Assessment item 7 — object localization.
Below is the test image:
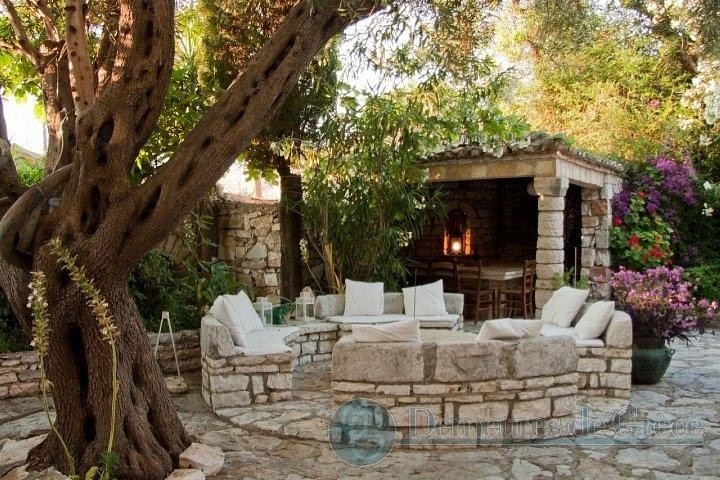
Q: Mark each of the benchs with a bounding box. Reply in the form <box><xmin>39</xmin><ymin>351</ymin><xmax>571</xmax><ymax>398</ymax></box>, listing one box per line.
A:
<box><xmin>329</xmin><ymin>322</ymin><xmax>582</xmax><ymax>424</ymax></box>
<box><xmin>201</xmin><ymin>290</ymin><xmax>302</xmax><ymax>410</ymax></box>
<box><xmin>537</xmin><ymin>293</ymin><xmax>635</xmax><ymax>399</ymax></box>
<box><xmin>317</xmin><ymin>277</ymin><xmax>466</xmax><ymax>335</ymax></box>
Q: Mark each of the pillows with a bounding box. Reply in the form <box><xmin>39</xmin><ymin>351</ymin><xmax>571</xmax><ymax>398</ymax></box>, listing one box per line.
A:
<box><xmin>572</xmin><ymin>301</ymin><xmax>617</xmax><ymax>339</ymax></box>
<box><xmin>542</xmin><ymin>282</ymin><xmax>589</xmax><ymax>327</ymax></box>
<box><xmin>402</xmin><ymin>278</ymin><xmax>450</xmax><ymax>316</ymax></box>
<box><xmin>341</xmin><ymin>274</ymin><xmax>385</xmax><ymax>319</ymax></box>
<box><xmin>208</xmin><ymin>293</ymin><xmax>248</xmax><ymax>347</ymax></box>
<box><xmin>349</xmin><ymin>318</ymin><xmax>425</xmax><ymax>343</ymax></box>
<box><xmin>475</xmin><ymin>314</ymin><xmax>545</xmax><ymax>341</ymax></box>
<box><xmin>222</xmin><ymin>289</ymin><xmax>262</xmax><ymax>332</ymax></box>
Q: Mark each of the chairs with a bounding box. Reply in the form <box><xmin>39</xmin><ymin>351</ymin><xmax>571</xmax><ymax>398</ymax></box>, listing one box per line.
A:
<box><xmin>409</xmin><ymin>252</ymin><xmax>536</xmax><ymax>320</ymax></box>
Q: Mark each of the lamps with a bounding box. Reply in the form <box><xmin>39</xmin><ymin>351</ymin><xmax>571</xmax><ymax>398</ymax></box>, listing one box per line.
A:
<box><xmin>444</xmin><ymin>226</ymin><xmax>468</xmax><ymax>257</ymax></box>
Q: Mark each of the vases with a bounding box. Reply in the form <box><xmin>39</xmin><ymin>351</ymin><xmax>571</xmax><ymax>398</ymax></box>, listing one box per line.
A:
<box><xmin>630</xmin><ymin>345</ymin><xmax>676</xmax><ymax>385</ymax></box>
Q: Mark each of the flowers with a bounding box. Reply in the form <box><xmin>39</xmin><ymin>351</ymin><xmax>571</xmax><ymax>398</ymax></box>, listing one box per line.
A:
<box><xmin>591</xmin><ymin>255</ymin><xmax>717</xmax><ymax>348</ymax></box>
<box><xmin>610</xmin><ymin>189</ymin><xmax>666</xmax><ymax>259</ymax></box>
